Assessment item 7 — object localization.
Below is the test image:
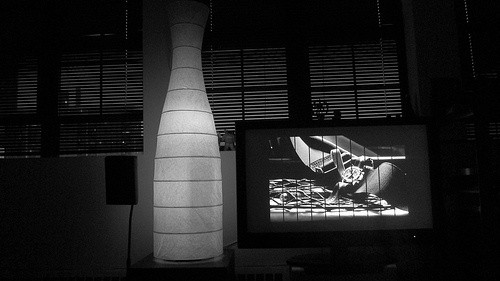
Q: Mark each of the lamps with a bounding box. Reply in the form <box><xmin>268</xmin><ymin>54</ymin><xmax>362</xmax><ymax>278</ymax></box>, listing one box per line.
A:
<box><xmin>151</xmin><ymin>1</ymin><xmax>224</xmax><ymax>262</ymax></box>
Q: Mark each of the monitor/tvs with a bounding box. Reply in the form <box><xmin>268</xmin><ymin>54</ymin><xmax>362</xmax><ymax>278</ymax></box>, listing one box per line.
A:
<box><xmin>236</xmin><ymin>116</ymin><xmax>445</xmax><ymax>249</ymax></box>
